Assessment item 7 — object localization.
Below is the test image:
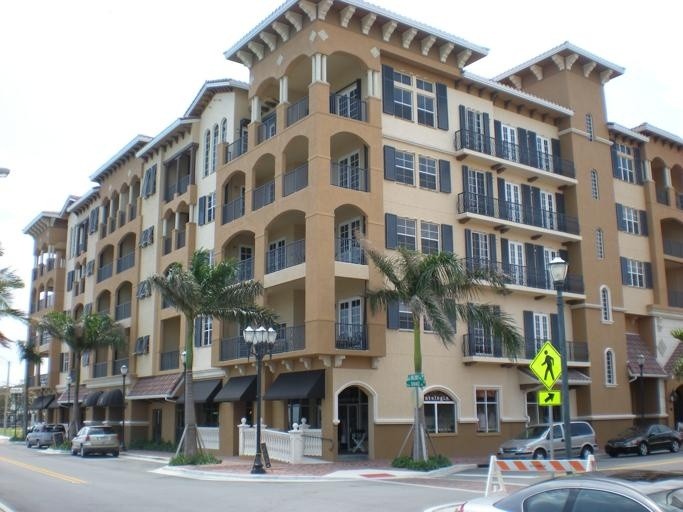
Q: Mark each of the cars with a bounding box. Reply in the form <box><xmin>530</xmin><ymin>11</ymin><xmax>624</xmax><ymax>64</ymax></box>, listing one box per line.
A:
<box><xmin>421</xmin><ymin>468</ymin><xmax>683</xmax><ymax>512</ymax></box>
<box><xmin>604</xmin><ymin>422</ymin><xmax>682</xmax><ymax>457</ymax></box>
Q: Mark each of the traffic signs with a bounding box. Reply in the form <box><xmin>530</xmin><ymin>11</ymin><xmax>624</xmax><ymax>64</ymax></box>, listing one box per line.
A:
<box><xmin>406</xmin><ymin>380</ymin><xmax>426</xmax><ymax>387</ymax></box>
<box><xmin>407</xmin><ymin>373</ymin><xmax>423</xmax><ymax>380</ymax></box>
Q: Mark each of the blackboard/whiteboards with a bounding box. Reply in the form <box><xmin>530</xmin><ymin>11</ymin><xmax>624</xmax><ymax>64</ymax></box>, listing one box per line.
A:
<box><xmin>52</xmin><ymin>432</ymin><xmax>64</xmax><ymax>448</ymax></box>
<box><xmin>260</xmin><ymin>442</ymin><xmax>271</xmax><ymax>468</ymax></box>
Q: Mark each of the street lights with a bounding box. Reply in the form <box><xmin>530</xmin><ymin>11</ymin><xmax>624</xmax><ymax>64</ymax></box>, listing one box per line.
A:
<box><xmin>242</xmin><ymin>324</ymin><xmax>277</xmax><ymax>473</ymax></box>
<box><xmin>636</xmin><ymin>353</ymin><xmax>645</xmax><ymax>422</ymax></box>
<box><xmin>546</xmin><ymin>255</ymin><xmax>576</xmax><ymax>476</ymax></box>
<box><xmin>120</xmin><ymin>364</ymin><xmax>128</xmax><ymax>449</ymax></box>
<box><xmin>40</xmin><ymin>381</ymin><xmax>44</xmax><ymax>423</ymax></box>
<box><xmin>65</xmin><ymin>376</ymin><xmax>70</xmax><ymax>440</ymax></box>
<box><xmin>180</xmin><ymin>350</ymin><xmax>186</xmax><ymax>408</ymax></box>
<box><xmin>13</xmin><ymin>389</ymin><xmax>17</xmax><ymax>439</ymax></box>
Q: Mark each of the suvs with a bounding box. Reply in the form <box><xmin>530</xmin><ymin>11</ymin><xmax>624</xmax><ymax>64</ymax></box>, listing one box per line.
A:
<box><xmin>70</xmin><ymin>425</ymin><xmax>120</xmax><ymax>457</ymax></box>
<box><xmin>23</xmin><ymin>423</ymin><xmax>64</xmax><ymax>448</ymax></box>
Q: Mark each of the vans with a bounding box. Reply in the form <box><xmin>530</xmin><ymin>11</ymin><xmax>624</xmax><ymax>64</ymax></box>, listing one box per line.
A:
<box><xmin>497</xmin><ymin>419</ymin><xmax>599</xmax><ymax>460</ymax></box>
